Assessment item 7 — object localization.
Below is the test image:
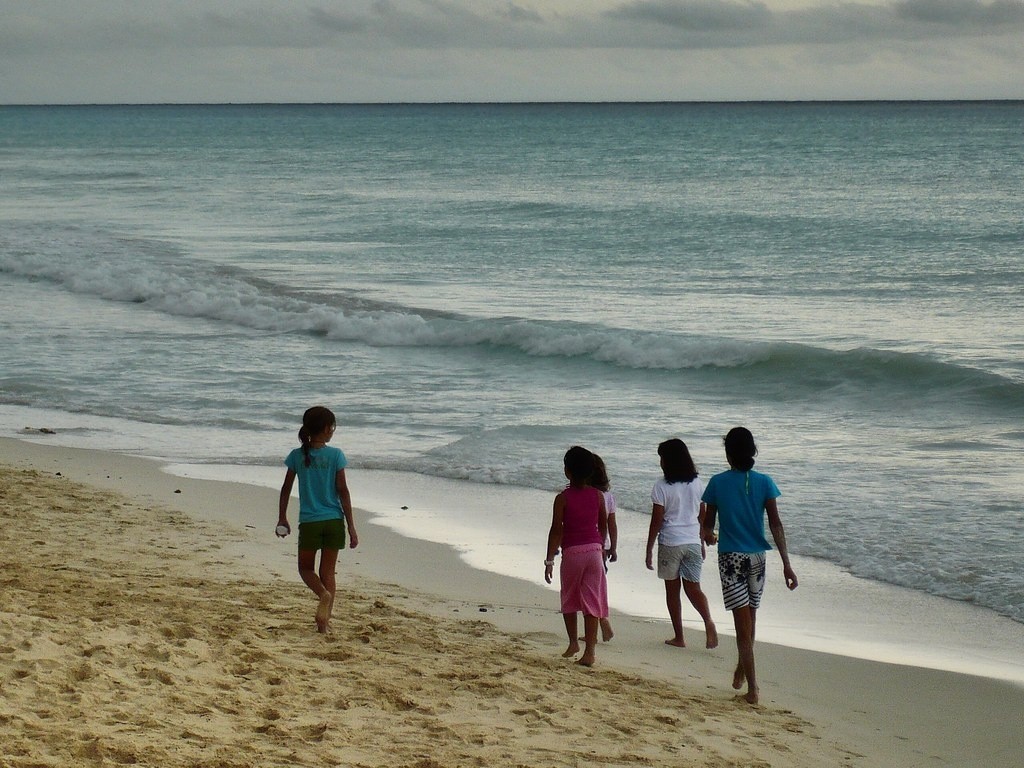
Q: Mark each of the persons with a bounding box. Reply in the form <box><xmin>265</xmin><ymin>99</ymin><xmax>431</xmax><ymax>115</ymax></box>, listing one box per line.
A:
<box><xmin>275</xmin><ymin>405</ymin><xmax>359</xmax><ymax>635</ymax></box>
<box><xmin>700</xmin><ymin>426</ymin><xmax>799</xmax><ymax>706</ymax></box>
<box><xmin>545</xmin><ymin>446</ymin><xmax>619</xmax><ymax>667</ymax></box>
<box><xmin>644</xmin><ymin>438</ymin><xmax>720</xmax><ymax>651</ymax></box>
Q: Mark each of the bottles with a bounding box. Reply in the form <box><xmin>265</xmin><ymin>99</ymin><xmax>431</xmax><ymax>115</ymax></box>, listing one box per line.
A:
<box><xmin>275</xmin><ymin>526</ymin><xmax>290</xmax><ymax>535</ymax></box>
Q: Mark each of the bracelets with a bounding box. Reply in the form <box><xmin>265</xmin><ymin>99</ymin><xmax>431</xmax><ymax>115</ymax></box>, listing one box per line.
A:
<box><xmin>544</xmin><ymin>560</ymin><xmax>555</xmax><ymax>565</ymax></box>
